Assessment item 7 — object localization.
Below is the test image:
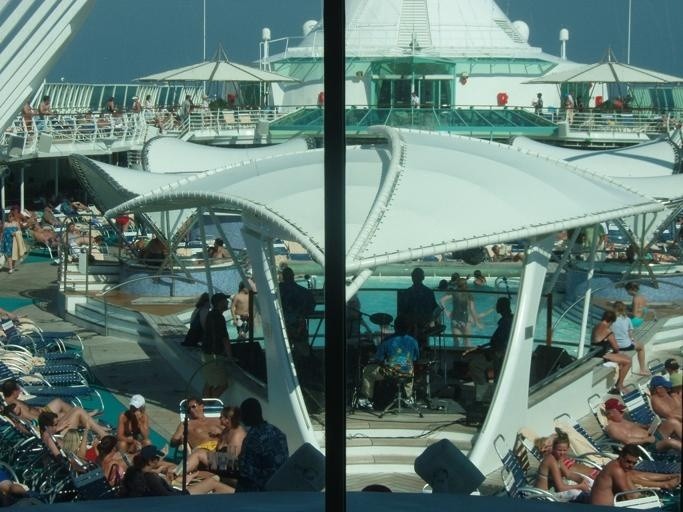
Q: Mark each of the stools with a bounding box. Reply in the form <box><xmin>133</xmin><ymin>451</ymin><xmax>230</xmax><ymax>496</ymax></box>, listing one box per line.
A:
<box><xmin>378</xmin><ymin>374</ymin><xmax>424</xmax><ymax>421</ymax></box>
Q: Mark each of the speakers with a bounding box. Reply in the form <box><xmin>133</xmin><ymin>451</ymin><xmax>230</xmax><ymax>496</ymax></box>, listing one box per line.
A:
<box><xmin>529</xmin><ymin>345</ymin><xmax>573</xmax><ymax>387</ymax></box>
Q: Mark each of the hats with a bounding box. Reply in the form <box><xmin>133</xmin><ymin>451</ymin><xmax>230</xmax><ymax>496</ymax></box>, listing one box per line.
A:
<box><xmin>664</xmin><ymin>358</ymin><xmax>680</xmax><ymax>371</ymax></box>
<box><xmin>130</xmin><ymin>394</ymin><xmax>146</xmax><ymax>408</ymax></box>
<box><xmin>141</xmin><ymin>446</ymin><xmax>166</xmax><ymax>460</ymax></box>
<box><xmin>650</xmin><ymin>376</ymin><xmax>673</xmax><ymax>389</ymax></box>
<box><xmin>604</xmin><ymin>399</ymin><xmax>627</xmax><ymax>411</ymax></box>
<box><xmin>211</xmin><ymin>293</ymin><xmax>230</xmax><ymax>303</ymax></box>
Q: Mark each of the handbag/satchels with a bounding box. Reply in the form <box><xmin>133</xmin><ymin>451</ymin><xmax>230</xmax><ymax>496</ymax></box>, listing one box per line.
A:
<box><xmin>180</xmin><ymin>329</ymin><xmax>202</xmax><ymax>347</ymax></box>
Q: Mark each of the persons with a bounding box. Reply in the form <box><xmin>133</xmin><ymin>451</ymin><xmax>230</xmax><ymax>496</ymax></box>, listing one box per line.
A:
<box><xmin>1</xmin><ymin>308</ymin><xmax>290</xmax><ymax>503</ymax></box>
<box><xmin>538</xmin><ymin>222</ymin><xmax>676</xmax><ymax>262</ymax></box>
<box><xmin>185</xmin><ymin>244</ymin><xmax>513</xmax><ymax>402</ymax></box>
<box><xmin>316</xmin><ymin>91</ymin><xmax>325</xmax><ymax>109</ymax></box>
<box><xmin>531</xmin><ymin>91</ymin><xmax>544</xmax><ymax>115</ymax></box>
<box><xmin>408</xmin><ymin>93</ymin><xmax>421</xmax><ymax>109</ymax></box>
<box><xmin>1</xmin><ymin>191</ymin><xmax>229</xmax><ymax>273</ymax></box>
<box><xmin>590</xmin><ymin>282</ymin><xmax>648</xmax><ymax>390</ymax></box>
<box><xmin>537</xmin><ymin>358</ymin><xmax>683</xmax><ymax>506</ymax></box>
<box><xmin>22</xmin><ymin>91</ymin><xmax>259</xmax><ymax>137</ymax></box>
<box><xmin>561</xmin><ymin>88</ymin><xmax>631</xmax><ymax>126</ymax></box>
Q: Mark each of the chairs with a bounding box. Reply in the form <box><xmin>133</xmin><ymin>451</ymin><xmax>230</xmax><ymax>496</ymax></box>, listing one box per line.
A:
<box><xmin>22</xmin><ymin>113</ymin><xmax>125</xmax><ymax>140</ymax></box>
<box><xmin>12</xmin><ymin>205</ymin><xmax>141</xmax><ymax>269</ymax></box>
<box><xmin>1</xmin><ymin>317</ymin><xmax>127</xmax><ymax>512</ymax></box>
<box><xmin>544</xmin><ymin>106</ymin><xmax>634</xmax><ymax>129</ymax></box>
<box><xmin>174</xmin><ymin>394</ymin><xmax>228</xmax><ymax>461</ymax></box>
<box><xmin>488</xmin><ymin>355</ymin><xmax>683</xmax><ymax>512</ymax></box>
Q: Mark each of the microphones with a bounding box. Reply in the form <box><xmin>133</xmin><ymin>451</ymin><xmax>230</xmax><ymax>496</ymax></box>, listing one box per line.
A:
<box><xmin>305</xmin><ymin>274</ymin><xmax>312</xmax><ymax>290</ymax></box>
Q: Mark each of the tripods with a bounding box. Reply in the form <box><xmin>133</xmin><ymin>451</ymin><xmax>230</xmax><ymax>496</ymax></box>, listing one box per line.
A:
<box><xmin>350</xmin><ymin>321</ymin><xmax>444</xmax><ymax>410</ymax></box>
